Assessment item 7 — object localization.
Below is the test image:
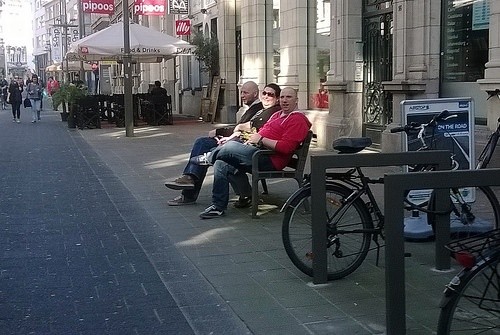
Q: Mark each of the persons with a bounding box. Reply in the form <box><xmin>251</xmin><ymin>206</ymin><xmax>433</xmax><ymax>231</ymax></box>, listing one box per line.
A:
<box><xmin>189</xmin><ymin>87</ymin><xmax>312</xmax><ymax>219</ymax></box>
<box><xmin>165</xmin><ymin>81</ymin><xmax>264</xmax><ymax>206</ymax></box>
<box><xmin>26</xmin><ymin>77</ymin><xmax>32</xmax><ymax>86</ymax></box>
<box><xmin>0</xmin><ymin>75</ymin><xmax>9</xmax><ymax>110</ymax></box>
<box><xmin>8</xmin><ymin>75</ymin><xmax>24</xmax><ymax>123</ymax></box>
<box><xmin>228</xmin><ymin>83</ymin><xmax>281</xmax><ymax>209</ymax></box>
<box><xmin>47</xmin><ymin>77</ymin><xmax>60</xmax><ymax>111</ymax></box>
<box><xmin>26</xmin><ymin>75</ymin><xmax>42</xmax><ymax>123</ymax></box>
<box><xmin>10</xmin><ymin>77</ymin><xmax>13</xmax><ymax>82</ymax></box>
<box><xmin>70</xmin><ymin>75</ymin><xmax>87</xmax><ymax>90</ymax></box>
<box><xmin>151</xmin><ymin>81</ymin><xmax>167</xmax><ymax>96</ymax></box>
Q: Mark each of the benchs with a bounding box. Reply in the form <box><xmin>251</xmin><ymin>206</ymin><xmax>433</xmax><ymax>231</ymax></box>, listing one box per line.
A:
<box><xmin>249</xmin><ymin>130</ymin><xmax>315</xmax><ymax>220</ymax></box>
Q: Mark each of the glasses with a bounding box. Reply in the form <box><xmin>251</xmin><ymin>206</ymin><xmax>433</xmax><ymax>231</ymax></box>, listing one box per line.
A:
<box><xmin>262</xmin><ymin>92</ymin><xmax>277</xmax><ymax>97</ymax></box>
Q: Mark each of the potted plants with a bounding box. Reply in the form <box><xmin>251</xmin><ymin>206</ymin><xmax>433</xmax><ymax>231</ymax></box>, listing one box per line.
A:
<box><xmin>52</xmin><ymin>80</ymin><xmax>85</xmax><ymax>128</ymax></box>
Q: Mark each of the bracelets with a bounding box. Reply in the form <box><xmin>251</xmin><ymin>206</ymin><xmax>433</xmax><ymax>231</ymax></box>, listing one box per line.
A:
<box><xmin>257</xmin><ymin>136</ymin><xmax>264</xmax><ymax>145</ymax></box>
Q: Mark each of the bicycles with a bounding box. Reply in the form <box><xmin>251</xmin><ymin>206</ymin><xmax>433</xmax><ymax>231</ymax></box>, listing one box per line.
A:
<box><xmin>434</xmin><ymin>228</ymin><xmax>500</xmax><ymax>335</ymax></box>
<box><xmin>279</xmin><ymin>108</ymin><xmax>500</xmax><ymax>280</ymax></box>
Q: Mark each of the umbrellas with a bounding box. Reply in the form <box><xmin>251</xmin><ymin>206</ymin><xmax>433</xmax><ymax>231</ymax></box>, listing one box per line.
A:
<box><xmin>65</xmin><ymin>17</ymin><xmax>200</xmax><ymax>63</ymax></box>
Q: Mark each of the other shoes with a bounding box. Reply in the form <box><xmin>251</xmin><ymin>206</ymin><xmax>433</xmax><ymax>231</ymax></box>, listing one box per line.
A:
<box><xmin>31</xmin><ymin>119</ymin><xmax>41</xmax><ymax>122</ymax></box>
<box><xmin>13</xmin><ymin>118</ymin><xmax>21</xmax><ymax>123</ymax></box>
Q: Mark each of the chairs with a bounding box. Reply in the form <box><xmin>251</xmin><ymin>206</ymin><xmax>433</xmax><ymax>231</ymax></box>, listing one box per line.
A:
<box><xmin>70</xmin><ymin>92</ymin><xmax>173</xmax><ymax>130</ymax></box>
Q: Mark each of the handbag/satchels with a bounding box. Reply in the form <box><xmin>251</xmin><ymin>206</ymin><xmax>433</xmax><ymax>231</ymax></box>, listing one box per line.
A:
<box><xmin>23</xmin><ymin>99</ymin><xmax>31</xmax><ymax>108</ymax></box>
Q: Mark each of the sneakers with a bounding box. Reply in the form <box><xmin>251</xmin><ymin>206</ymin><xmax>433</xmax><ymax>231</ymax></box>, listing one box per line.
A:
<box><xmin>165</xmin><ymin>175</ymin><xmax>194</xmax><ymax>190</ymax></box>
<box><xmin>168</xmin><ymin>194</ymin><xmax>197</xmax><ymax>205</ymax></box>
<box><xmin>234</xmin><ymin>197</ymin><xmax>252</xmax><ymax>208</ymax></box>
<box><xmin>199</xmin><ymin>205</ymin><xmax>226</xmax><ymax>218</ymax></box>
